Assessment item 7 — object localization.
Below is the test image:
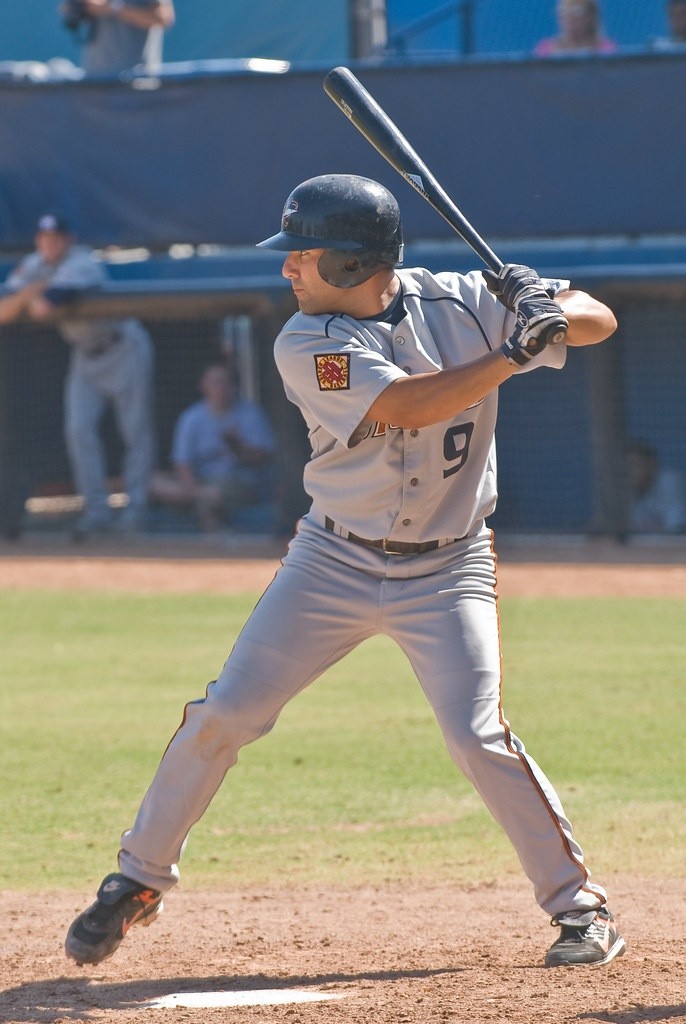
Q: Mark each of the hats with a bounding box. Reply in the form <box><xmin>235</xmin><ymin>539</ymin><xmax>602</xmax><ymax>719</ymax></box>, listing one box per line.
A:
<box><xmin>31</xmin><ymin>211</ymin><xmax>71</xmax><ymax>241</ymax></box>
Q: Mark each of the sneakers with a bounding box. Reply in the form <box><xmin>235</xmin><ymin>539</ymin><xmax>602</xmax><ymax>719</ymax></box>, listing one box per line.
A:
<box><xmin>544</xmin><ymin>904</ymin><xmax>626</xmax><ymax>969</ymax></box>
<box><xmin>65</xmin><ymin>873</ymin><xmax>163</xmax><ymax>966</ymax></box>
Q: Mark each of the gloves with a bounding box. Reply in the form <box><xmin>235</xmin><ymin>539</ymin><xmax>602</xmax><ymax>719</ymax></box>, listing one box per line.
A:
<box><xmin>482</xmin><ymin>263</ymin><xmax>556</xmax><ymax>314</ymax></box>
<box><xmin>500</xmin><ymin>296</ymin><xmax>569</xmax><ymax>368</ymax></box>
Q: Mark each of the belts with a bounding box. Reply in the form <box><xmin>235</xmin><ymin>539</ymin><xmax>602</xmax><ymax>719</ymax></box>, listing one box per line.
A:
<box><xmin>325</xmin><ymin>516</ymin><xmax>467</xmax><ymax>555</ymax></box>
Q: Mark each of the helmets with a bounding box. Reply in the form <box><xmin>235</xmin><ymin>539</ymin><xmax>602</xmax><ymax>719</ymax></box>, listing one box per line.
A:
<box><xmin>255</xmin><ymin>174</ymin><xmax>404</xmax><ymax>289</ymax></box>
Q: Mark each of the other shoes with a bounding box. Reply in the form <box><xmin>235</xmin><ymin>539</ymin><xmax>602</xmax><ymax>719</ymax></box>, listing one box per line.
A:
<box><xmin>73</xmin><ymin>505</ymin><xmax>111</xmax><ymax>540</ymax></box>
<box><xmin>117</xmin><ymin>500</ymin><xmax>147</xmax><ymax>537</ymax></box>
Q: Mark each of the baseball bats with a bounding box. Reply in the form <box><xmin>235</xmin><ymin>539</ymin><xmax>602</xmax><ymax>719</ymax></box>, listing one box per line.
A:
<box><xmin>324</xmin><ymin>66</ymin><xmax>568</xmax><ymax>346</ymax></box>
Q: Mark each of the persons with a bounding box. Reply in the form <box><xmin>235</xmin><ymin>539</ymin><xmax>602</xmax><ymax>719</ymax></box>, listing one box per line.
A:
<box><xmin>63</xmin><ymin>0</ymin><xmax>175</xmax><ymax>69</ymax></box>
<box><xmin>0</xmin><ymin>210</ymin><xmax>294</xmax><ymax>541</ymax></box>
<box><xmin>619</xmin><ymin>438</ymin><xmax>686</xmax><ymax>537</ymax></box>
<box><xmin>535</xmin><ymin>0</ymin><xmax>686</xmax><ymax>54</ymax></box>
<box><xmin>66</xmin><ymin>173</ymin><xmax>626</xmax><ymax>967</ymax></box>
